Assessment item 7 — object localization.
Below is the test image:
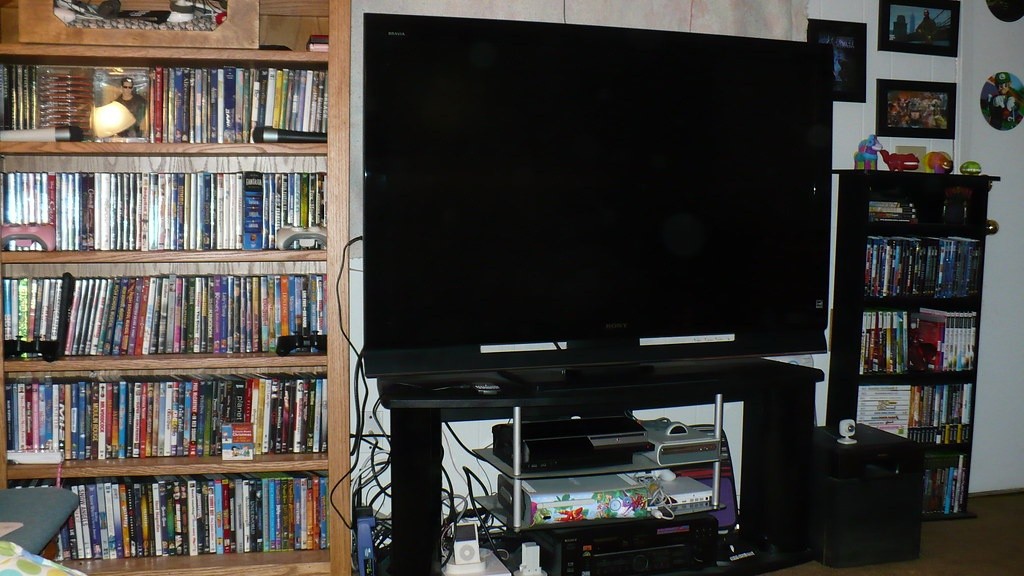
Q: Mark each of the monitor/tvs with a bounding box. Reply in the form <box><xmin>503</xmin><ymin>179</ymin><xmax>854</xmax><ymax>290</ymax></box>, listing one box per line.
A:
<box><xmin>362</xmin><ymin>11</ymin><xmax>835</xmax><ymax>396</ymax></box>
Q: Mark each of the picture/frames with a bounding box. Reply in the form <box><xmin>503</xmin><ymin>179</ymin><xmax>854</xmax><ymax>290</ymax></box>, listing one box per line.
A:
<box><xmin>874</xmin><ymin>79</ymin><xmax>956</xmax><ymax>141</ymax></box>
<box><xmin>876</xmin><ymin>0</ymin><xmax>961</xmax><ymax>58</ymax></box>
<box><xmin>806</xmin><ymin>18</ymin><xmax>867</xmax><ymax>103</ymax></box>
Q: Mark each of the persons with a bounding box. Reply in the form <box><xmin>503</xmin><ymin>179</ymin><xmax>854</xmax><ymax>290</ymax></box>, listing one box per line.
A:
<box><xmin>112</xmin><ymin>77</ymin><xmax>147</xmax><ymax>138</ymax></box>
<box><xmin>917</xmin><ymin>9</ymin><xmax>936</xmax><ymax>45</ymax></box>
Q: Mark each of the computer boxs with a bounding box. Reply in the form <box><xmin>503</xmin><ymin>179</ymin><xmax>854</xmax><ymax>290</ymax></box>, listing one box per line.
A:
<box><xmin>812</xmin><ymin>423</ymin><xmax>924</xmax><ymax>569</ymax></box>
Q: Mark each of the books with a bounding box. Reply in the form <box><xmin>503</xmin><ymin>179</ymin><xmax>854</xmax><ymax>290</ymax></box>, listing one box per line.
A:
<box><xmin>0</xmin><ymin>34</ymin><xmax>328</xmax><ymax>144</ymax></box>
<box><xmin>5</xmin><ymin>371</ymin><xmax>329</xmax><ymax>465</ymax></box>
<box><xmin>8</xmin><ymin>469</ymin><xmax>331</xmax><ymax>562</ymax></box>
<box><xmin>2</xmin><ymin>272</ymin><xmax>328</xmax><ymax>359</ymax></box>
<box><xmin>0</xmin><ymin>171</ymin><xmax>328</xmax><ymax>251</ymax></box>
<box><xmin>859</xmin><ymin>307</ymin><xmax>977</xmax><ymax>375</ymax></box>
<box><xmin>856</xmin><ymin>383</ymin><xmax>972</xmax><ymax>444</ymax></box>
<box><xmin>864</xmin><ymin>235</ymin><xmax>981</xmax><ymax>299</ymax></box>
<box><xmin>868</xmin><ymin>201</ymin><xmax>918</xmax><ymax>224</ymax></box>
<box><xmin>921</xmin><ymin>454</ymin><xmax>966</xmax><ymax>514</ymax></box>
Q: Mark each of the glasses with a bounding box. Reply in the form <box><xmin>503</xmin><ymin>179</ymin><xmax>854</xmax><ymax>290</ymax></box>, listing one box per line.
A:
<box><xmin>123</xmin><ymin>86</ymin><xmax>132</xmax><ymax>89</ymax></box>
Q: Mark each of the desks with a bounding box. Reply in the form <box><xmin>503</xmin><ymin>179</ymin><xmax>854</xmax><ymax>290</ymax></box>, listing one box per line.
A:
<box><xmin>374</xmin><ymin>353</ymin><xmax>825</xmax><ymax>576</ymax></box>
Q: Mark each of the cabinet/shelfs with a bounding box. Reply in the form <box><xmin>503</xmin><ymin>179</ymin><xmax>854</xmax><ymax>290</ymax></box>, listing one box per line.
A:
<box><xmin>825</xmin><ymin>166</ymin><xmax>1003</xmax><ymax>522</ymax></box>
<box><xmin>0</xmin><ymin>0</ymin><xmax>351</xmax><ymax>576</ymax></box>
